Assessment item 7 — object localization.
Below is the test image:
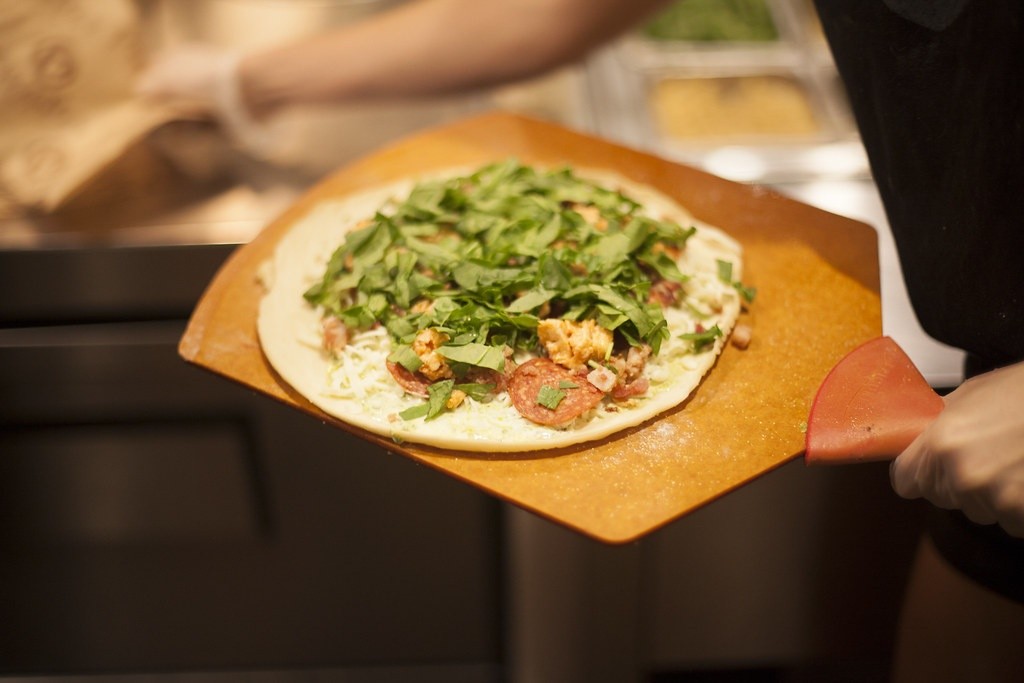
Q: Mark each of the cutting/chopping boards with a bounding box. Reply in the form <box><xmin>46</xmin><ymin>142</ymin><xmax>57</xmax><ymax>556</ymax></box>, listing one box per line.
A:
<box><xmin>177</xmin><ymin>112</ymin><xmax>942</xmax><ymax>541</ymax></box>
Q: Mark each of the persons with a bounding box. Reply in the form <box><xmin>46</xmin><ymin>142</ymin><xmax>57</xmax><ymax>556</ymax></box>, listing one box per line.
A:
<box><xmin>137</xmin><ymin>0</ymin><xmax>1024</xmax><ymax>683</ymax></box>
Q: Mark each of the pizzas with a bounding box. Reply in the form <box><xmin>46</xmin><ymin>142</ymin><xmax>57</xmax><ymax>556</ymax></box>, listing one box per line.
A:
<box><xmin>254</xmin><ymin>158</ymin><xmax>755</xmax><ymax>453</ymax></box>
